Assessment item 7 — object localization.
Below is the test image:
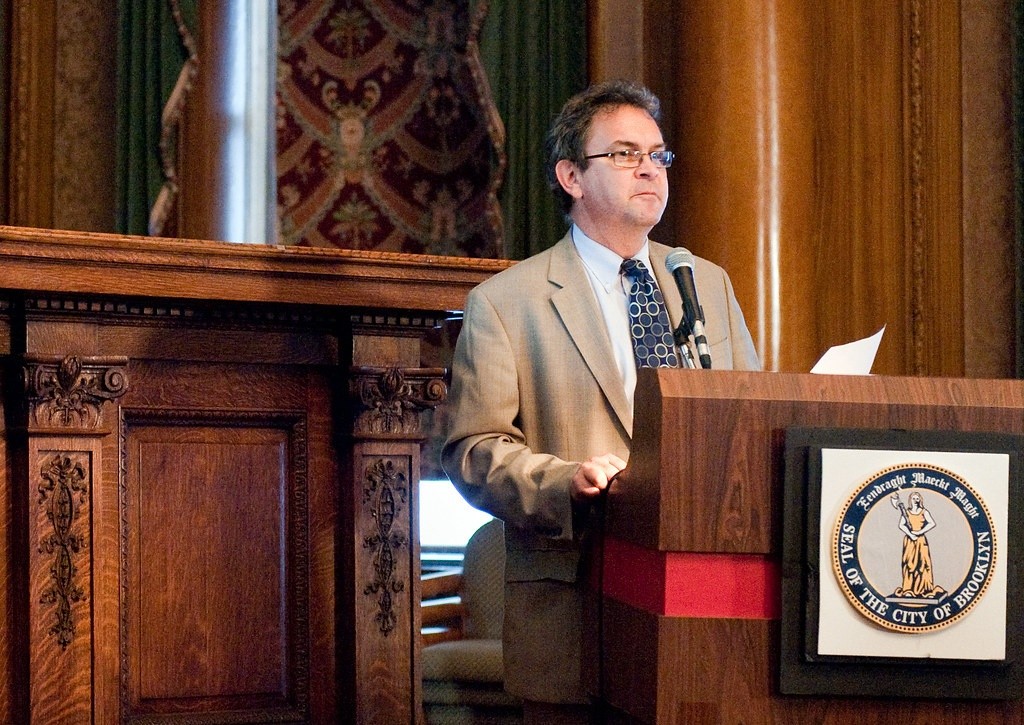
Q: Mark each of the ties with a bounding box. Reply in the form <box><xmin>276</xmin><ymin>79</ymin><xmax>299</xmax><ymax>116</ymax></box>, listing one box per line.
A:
<box><xmin>622</xmin><ymin>259</ymin><xmax>678</xmax><ymax>371</ymax></box>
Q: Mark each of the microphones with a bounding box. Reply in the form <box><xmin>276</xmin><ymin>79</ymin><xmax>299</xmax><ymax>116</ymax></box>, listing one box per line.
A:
<box><xmin>665</xmin><ymin>246</ymin><xmax>712</xmax><ymax>369</ymax></box>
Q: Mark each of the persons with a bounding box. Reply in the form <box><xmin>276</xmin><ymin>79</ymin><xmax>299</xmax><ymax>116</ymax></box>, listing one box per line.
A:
<box><xmin>442</xmin><ymin>76</ymin><xmax>761</xmax><ymax>725</ymax></box>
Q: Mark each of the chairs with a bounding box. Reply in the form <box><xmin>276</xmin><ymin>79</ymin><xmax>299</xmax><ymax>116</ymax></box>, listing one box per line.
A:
<box><xmin>421</xmin><ymin>517</ymin><xmax>524</xmax><ymax>725</ymax></box>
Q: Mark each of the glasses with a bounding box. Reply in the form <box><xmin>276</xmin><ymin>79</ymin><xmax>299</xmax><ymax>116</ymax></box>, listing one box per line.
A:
<box><xmin>583</xmin><ymin>150</ymin><xmax>675</xmax><ymax>168</ymax></box>
